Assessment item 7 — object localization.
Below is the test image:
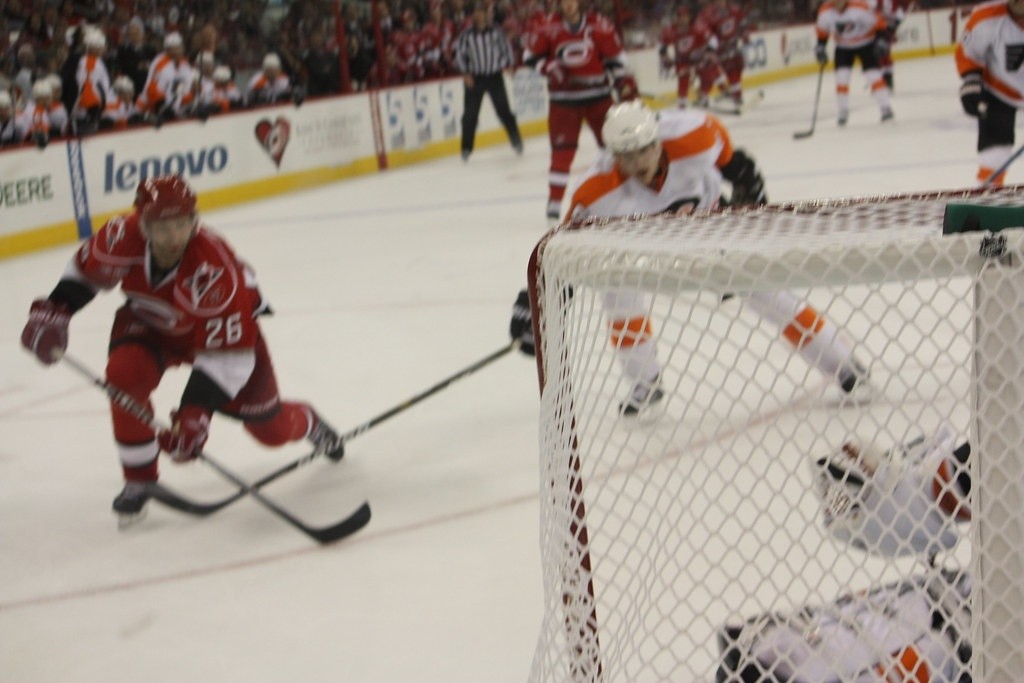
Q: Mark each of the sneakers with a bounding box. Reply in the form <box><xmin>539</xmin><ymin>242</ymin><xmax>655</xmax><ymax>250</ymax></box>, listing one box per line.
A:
<box><xmin>114</xmin><ymin>478</ymin><xmax>157</xmax><ymax>531</ymax></box>
<box><xmin>305</xmin><ymin>409</ymin><xmax>344</xmax><ymax>462</ymax></box>
<box><xmin>547</xmin><ymin>198</ymin><xmax>560</xmax><ymax>222</ymax></box>
<box><xmin>618</xmin><ymin>376</ymin><xmax>666</xmax><ymax>427</ymax></box>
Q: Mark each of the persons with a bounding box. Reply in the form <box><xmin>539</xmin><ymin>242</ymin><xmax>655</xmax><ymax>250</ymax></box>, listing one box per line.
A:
<box><xmin>455</xmin><ymin>6</ymin><xmax>527</xmax><ymax>163</ymax></box>
<box><xmin>954</xmin><ymin>0</ymin><xmax>1024</xmax><ymax>266</ymax></box>
<box><xmin>0</xmin><ymin>0</ymin><xmax>342</xmax><ymax>150</ymax></box>
<box><xmin>510</xmin><ymin>98</ymin><xmax>873</xmax><ymax>426</ymax></box>
<box><xmin>814</xmin><ymin>0</ymin><xmax>906</xmax><ymax>128</ymax></box>
<box><xmin>658</xmin><ymin>0</ymin><xmax>751</xmax><ymax>115</ymax></box>
<box><xmin>712</xmin><ymin>433</ymin><xmax>972</xmax><ymax>683</ymax></box>
<box><xmin>20</xmin><ymin>178</ymin><xmax>345</xmax><ymax>531</ymax></box>
<box><xmin>522</xmin><ymin>0</ymin><xmax>642</xmax><ymax>228</ymax></box>
<box><xmin>345</xmin><ymin>1</ymin><xmax>619</xmax><ymax>85</ymax></box>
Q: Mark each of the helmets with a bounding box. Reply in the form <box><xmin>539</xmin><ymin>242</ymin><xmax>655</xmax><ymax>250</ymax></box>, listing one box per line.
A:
<box><xmin>600</xmin><ymin>100</ymin><xmax>656</xmax><ymax>156</ymax></box>
<box><xmin>32</xmin><ymin>28</ymin><xmax>284</xmax><ymax>101</ymax></box>
<box><xmin>135</xmin><ymin>175</ymin><xmax>195</xmax><ymax>219</ymax></box>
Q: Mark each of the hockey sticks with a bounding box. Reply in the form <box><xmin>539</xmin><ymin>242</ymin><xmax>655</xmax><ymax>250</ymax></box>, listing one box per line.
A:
<box><xmin>145</xmin><ymin>342</ymin><xmax>519</xmax><ymax>517</ymax></box>
<box><xmin>793</xmin><ymin>60</ymin><xmax>827</xmax><ymax>141</ymax></box>
<box><xmin>51</xmin><ymin>347</ymin><xmax>373</xmax><ymax>544</ymax></box>
<box><xmin>982</xmin><ymin>145</ymin><xmax>1024</xmax><ymax>187</ymax></box>
<box><xmin>565</xmin><ymin>74</ymin><xmax>766</xmax><ymax>117</ymax></box>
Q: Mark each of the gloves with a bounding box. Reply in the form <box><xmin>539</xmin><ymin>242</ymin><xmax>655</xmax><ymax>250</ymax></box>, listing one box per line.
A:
<box><xmin>961</xmin><ymin>71</ymin><xmax>989</xmax><ymax>121</ymax></box>
<box><xmin>815</xmin><ymin>40</ymin><xmax>826</xmax><ymax>64</ymax></box>
<box><xmin>876</xmin><ymin>32</ymin><xmax>891</xmax><ymax>55</ymax></box>
<box><xmin>731</xmin><ymin>173</ymin><xmax>767</xmax><ymax>208</ymax></box>
<box><xmin>21</xmin><ymin>299</ymin><xmax>72</xmax><ymax>368</ymax></box>
<box><xmin>511</xmin><ymin>291</ymin><xmax>537</xmax><ymax>355</ymax></box>
<box><xmin>157</xmin><ymin>406</ymin><xmax>213</xmax><ymax>462</ymax></box>
<box><xmin>613</xmin><ymin>73</ymin><xmax>637</xmax><ymax>100</ymax></box>
<box><xmin>542</xmin><ymin>59</ymin><xmax>567</xmax><ymax>88</ymax></box>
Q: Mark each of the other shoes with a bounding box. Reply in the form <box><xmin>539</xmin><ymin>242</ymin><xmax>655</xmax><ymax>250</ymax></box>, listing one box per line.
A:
<box><xmin>515</xmin><ymin>139</ymin><xmax>522</xmax><ymax>155</ymax></box>
<box><xmin>882</xmin><ymin>111</ymin><xmax>892</xmax><ymax>124</ymax></box>
<box><xmin>838</xmin><ymin>118</ymin><xmax>846</xmax><ymax>127</ymax></box>
<box><xmin>461</xmin><ymin>147</ymin><xmax>470</xmax><ymax>160</ymax></box>
